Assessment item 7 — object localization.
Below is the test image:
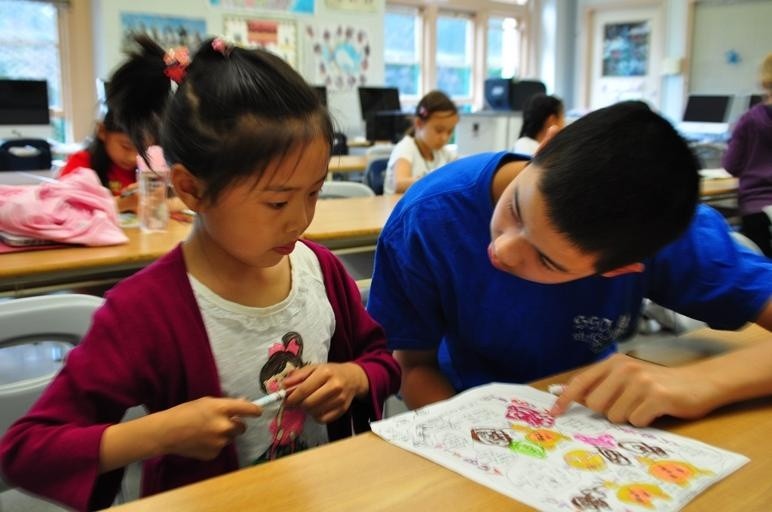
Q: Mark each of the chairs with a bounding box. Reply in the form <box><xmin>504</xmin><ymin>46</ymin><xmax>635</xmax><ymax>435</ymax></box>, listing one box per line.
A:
<box><xmin>359</xmin><ymin>87</ymin><xmax>404</xmax><ymax>141</ymax></box>
<box><xmin>364</xmin><ymin>156</ymin><xmax>390</xmax><ymax>194</ymax></box>
<box><xmin>308</xmin><ymin>179</ymin><xmax>374</xmax><ymax>201</ymax></box>
<box><xmin>0</xmin><ymin>292</ymin><xmax>152</xmax><ymax>512</ymax></box>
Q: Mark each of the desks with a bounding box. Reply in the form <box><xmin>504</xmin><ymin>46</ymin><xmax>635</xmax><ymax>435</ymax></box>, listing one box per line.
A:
<box><xmin>103</xmin><ymin>312</ymin><xmax>772</xmax><ymax>512</ymax></box>
<box><xmin>1</xmin><ymin>165</ymin><xmax>742</xmax><ymax>298</ymax></box>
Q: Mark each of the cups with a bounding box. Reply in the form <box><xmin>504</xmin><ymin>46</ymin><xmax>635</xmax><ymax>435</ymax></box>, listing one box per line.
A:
<box><xmin>137</xmin><ymin>169</ymin><xmax>170</xmax><ymax>234</ymax></box>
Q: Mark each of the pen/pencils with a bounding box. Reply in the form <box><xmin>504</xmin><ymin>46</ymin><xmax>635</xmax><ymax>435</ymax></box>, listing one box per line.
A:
<box><xmin>548</xmin><ymin>382</ymin><xmax>570</xmax><ymax>396</ymax></box>
<box><xmin>121</xmin><ymin>188</ymin><xmax>139</xmax><ymax>198</ymax></box>
<box><xmin>231</xmin><ymin>384</ymin><xmax>299</xmax><ymax>418</ymax></box>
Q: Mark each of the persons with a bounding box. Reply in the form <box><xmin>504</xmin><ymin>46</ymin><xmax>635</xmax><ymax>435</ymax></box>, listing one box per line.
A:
<box><xmin>366</xmin><ymin>99</ymin><xmax>772</xmax><ymax>429</ymax></box>
<box><xmin>515</xmin><ymin>95</ymin><xmax>565</xmax><ymax>158</ymax></box>
<box><xmin>722</xmin><ymin>55</ymin><xmax>772</xmax><ymax>259</ymax></box>
<box><xmin>0</xmin><ymin>35</ymin><xmax>402</xmax><ymax>510</ymax></box>
<box><xmin>383</xmin><ymin>89</ymin><xmax>458</xmax><ymax>196</ymax></box>
<box><xmin>59</xmin><ymin>107</ymin><xmax>191</xmax><ymax>220</ymax></box>
